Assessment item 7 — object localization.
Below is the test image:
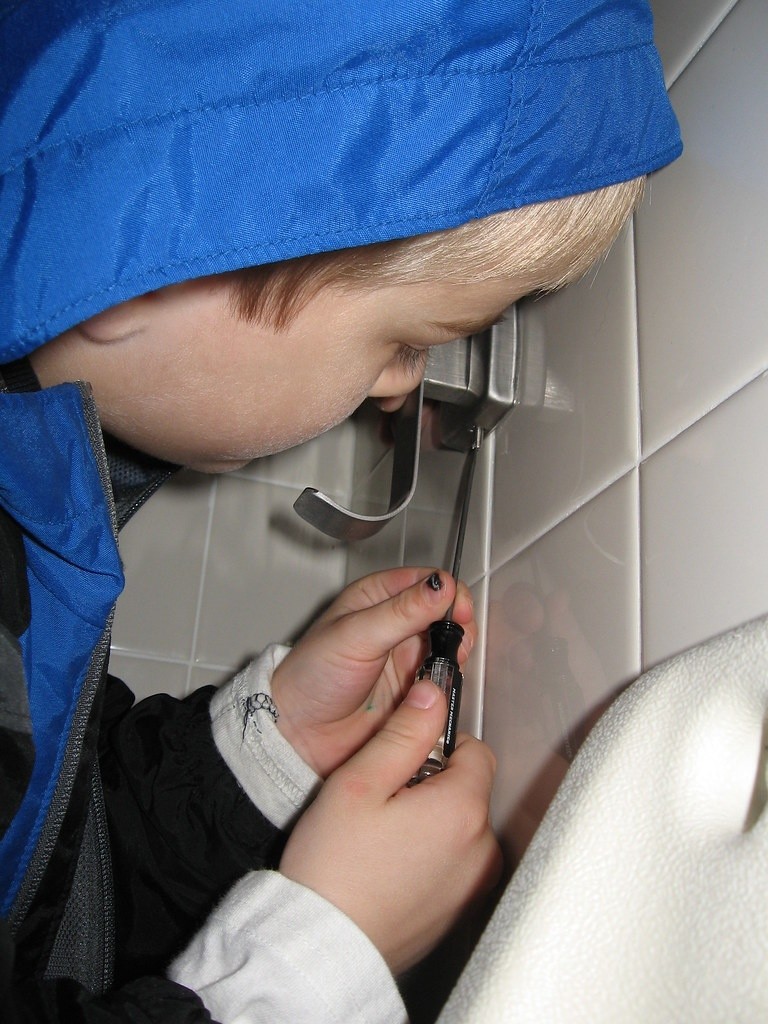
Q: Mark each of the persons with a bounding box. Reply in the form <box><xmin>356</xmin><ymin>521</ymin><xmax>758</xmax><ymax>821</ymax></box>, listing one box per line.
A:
<box><xmin>0</xmin><ymin>0</ymin><xmax>692</xmax><ymax>1024</ymax></box>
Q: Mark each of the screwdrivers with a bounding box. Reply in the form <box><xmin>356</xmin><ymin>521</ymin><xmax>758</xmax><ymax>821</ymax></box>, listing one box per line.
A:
<box><xmin>402</xmin><ymin>447</ymin><xmax>479</xmax><ymax>786</ymax></box>
